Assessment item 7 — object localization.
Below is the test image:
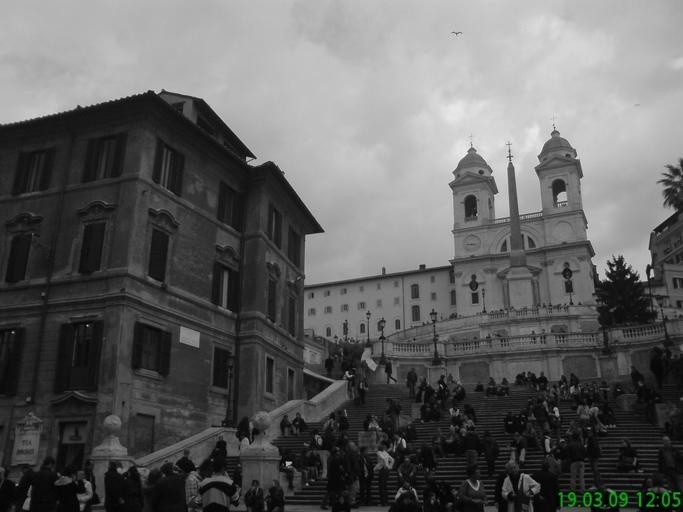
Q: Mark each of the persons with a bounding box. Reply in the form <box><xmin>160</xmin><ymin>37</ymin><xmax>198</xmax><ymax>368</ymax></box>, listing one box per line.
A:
<box><xmin>1</xmin><ymin>416</ymin><xmax>286</xmax><ymax>512</ymax></box>
<box><xmin>446</xmin><ymin>301</ymin><xmax>582</xmax><ymax>314</ymax></box>
<box><xmin>472</xmin><ymin>327</ymin><xmax>567</xmax><ymax>348</ymax></box>
<box><xmin>334</xmin><ymin>334</ymin><xmax>360</xmax><ymax>345</ymax></box>
<box><xmin>277</xmin><ymin>397</ymin><xmax>500</xmax><ymax>512</ymax></box>
<box><xmin>407</xmin><ymin>367</ymin><xmax>467</xmax><ymax>424</ymax></box>
<box><xmin>474</xmin><ymin>346</ymin><xmax>683</xmax><ymax>512</ymax></box>
<box><xmin>326</xmin><ymin>347</ymin><xmax>397</xmax><ymax>404</ymax></box>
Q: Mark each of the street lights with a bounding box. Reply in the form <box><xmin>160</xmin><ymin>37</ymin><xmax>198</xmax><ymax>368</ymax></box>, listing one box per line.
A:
<box><xmin>379</xmin><ymin>318</ymin><xmax>387</xmax><ymax>365</ymax></box>
<box><xmin>656</xmin><ymin>295</ymin><xmax>675</xmax><ymax>347</ymax></box>
<box><xmin>366</xmin><ymin>310</ymin><xmax>371</xmax><ymax>347</ymax></box>
<box><xmin>567</xmin><ymin>280</ymin><xmax>573</xmax><ymax>305</ymax></box>
<box><xmin>482</xmin><ymin>288</ymin><xmax>487</xmax><ymax>313</ymax></box>
<box><xmin>430</xmin><ymin>309</ymin><xmax>441</xmax><ymax>365</ymax></box>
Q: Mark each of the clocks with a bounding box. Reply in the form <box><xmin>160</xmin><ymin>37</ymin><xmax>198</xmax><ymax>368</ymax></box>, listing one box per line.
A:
<box><xmin>463</xmin><ymin>235</ymin><xmax>481</xmax><ymax>253</ymax></box>
